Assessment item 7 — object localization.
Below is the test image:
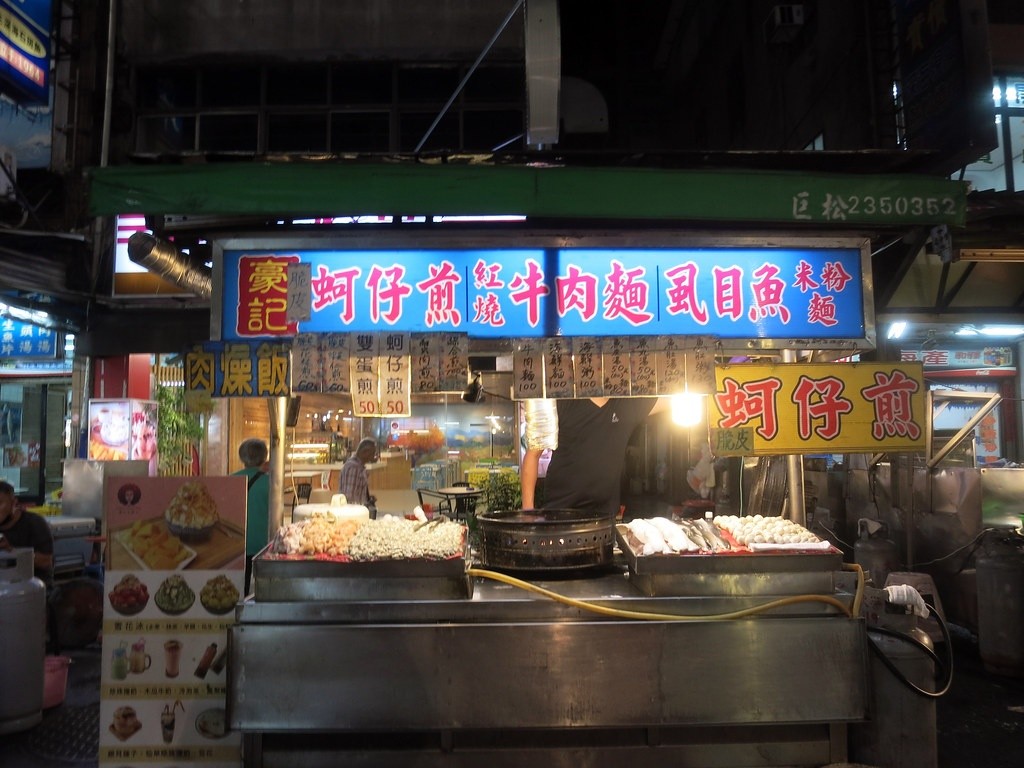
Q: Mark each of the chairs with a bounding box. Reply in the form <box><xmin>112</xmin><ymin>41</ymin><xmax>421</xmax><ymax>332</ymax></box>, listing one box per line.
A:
<box><xmin>417</xmin><ymin>482</ymin><xmax>477</xmax><ymax>521</ymax></box>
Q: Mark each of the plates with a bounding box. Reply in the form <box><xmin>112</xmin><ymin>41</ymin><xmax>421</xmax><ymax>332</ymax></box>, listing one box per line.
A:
<box><xmin>195</xmin><ymin>707</ymin><xmax>231</xmax><ymax>739</ymax></box>
<box><xmin>114</xmin><ymin>528</ymin><xmax>197</xmax><ymax>571</ymax></box>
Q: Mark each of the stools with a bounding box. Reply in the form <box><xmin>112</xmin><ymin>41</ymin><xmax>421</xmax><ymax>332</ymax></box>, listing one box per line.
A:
<box><xmin>885</xmin><ymin>572</ymin><xmax>947</xmax><ymax>643</ymax></box>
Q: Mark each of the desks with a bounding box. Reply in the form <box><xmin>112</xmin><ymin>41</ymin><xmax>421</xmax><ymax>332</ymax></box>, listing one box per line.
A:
<box><xmin>439</xmin><ymin>487</ymin><xmax>482</xmax><ymax>521</ymax></box>
<box><xmin>293</xmin><ymin>502</ymin><xmax>370</xmax><ymax>523</ymax></box>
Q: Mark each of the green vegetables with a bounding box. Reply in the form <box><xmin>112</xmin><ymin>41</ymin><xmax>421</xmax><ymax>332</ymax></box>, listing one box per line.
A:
<box><xmin>478</xmin><ymin>472</ymin><xmax>522</xmax><ymax>513</ymax></box>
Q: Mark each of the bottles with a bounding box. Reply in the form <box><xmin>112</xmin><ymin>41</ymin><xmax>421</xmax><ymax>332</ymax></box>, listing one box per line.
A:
<box><xmin>193</xmin><ymin>643</ymin><xmax>226</xmax><ymax>680</ymax></box>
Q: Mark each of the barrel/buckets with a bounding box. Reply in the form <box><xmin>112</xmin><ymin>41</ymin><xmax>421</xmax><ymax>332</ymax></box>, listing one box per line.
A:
<box><xmin>46</xmin><ymin>576</ymin><xmax>104</xmax><ymax>649</ymax></box>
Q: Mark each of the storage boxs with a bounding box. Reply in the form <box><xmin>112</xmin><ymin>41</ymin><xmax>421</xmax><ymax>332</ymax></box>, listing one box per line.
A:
<box><xmin>46</xmin><ymin>515</ymin><xmax>96</xmax><ymax>568</ymax></box>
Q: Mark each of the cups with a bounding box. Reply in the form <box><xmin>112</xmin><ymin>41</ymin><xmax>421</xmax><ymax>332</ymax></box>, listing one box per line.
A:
<box><xmin>43</xmin><ymin>656</ymin><xmax>71</xmax><ymax>709</ymax></box>
<box><xmin>110</xmin><ymin>640</ymin><xmax>151</xmax><ymax>681</ymax></box>
<box><xmin>161</xmin><ymin>713</ymin><xmax>174</xmax><ymax>743</ymax></box>
<box><xmin>163</xmin><ymin>640</ymin><xmax>183</xmax><ymax>677</ymax></box>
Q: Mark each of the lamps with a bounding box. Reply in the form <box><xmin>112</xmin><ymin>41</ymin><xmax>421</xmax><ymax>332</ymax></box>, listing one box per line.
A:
<box><xmin>461</xmin><ymin>370</ymin><xmax>510</xmax><ymax>404</ymax></box>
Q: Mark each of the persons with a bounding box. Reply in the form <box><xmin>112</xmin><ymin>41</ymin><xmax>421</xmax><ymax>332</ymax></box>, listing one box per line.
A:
<box><xmin>518</xmin><ymin>399</ymin><xmax>657</xmax><ymax>519</ymax></box>
<box><xmin>0</xmin><ymin>481</ymin><xmax>54</xmax><ymax>596</ymax></box>
<box><xmin>229</xmin><ymin>439</ymin><xmax>271</xmax><ymax>600</ymax></box>
<box><xmin>339</xmin><ymin>437</ymin><xmax>378</xmax><ymax>520</ymax></box>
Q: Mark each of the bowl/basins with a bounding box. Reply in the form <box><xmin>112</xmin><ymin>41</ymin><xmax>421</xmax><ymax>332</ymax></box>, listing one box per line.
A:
<box><xmin>108</xmin><ymin>592</ymin><xmax>149</xmax><ymax>616</ymax></box>
<box><xmin>154</xmin><ymin>590</ymin><xmax>196</xmax><ymax>615</ymax></box>
<box><xmin>165</xmin><ymin>514</ymin><xmax>219</xmax><ymax>542</ymax></box>
<box><xmin>201</xmin><ymin>594</ymin><xmax>239</xmax><ymax>615</ymax></box>
